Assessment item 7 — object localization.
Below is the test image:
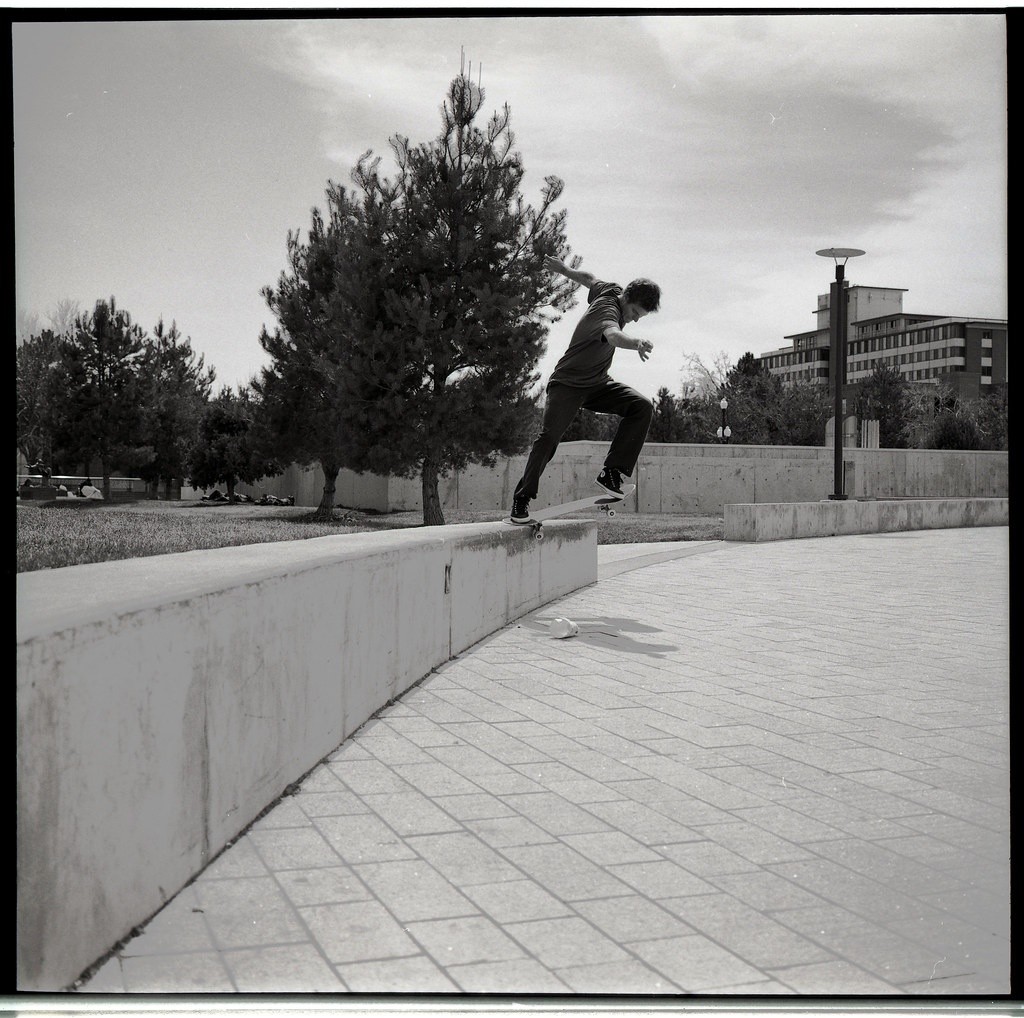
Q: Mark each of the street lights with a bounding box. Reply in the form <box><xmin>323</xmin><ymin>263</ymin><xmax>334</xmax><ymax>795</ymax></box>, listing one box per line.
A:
<box><xmin>816</xmin><ymin>248</ymin><xmax>866</xmax><ymax>501</ymax></box>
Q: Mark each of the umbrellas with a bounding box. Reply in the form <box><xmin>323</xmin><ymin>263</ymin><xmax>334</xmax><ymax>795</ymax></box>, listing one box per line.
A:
<box><xmin>82</xmin><ymin>486</ymin><xmax>102</xmax><ymax>499</ymax></box>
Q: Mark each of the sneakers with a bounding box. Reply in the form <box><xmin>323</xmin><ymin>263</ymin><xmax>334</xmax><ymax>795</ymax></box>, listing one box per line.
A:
<box><xmin>511</xmin><ymin>497</ymin><xmax>530</xmax><ymax>522</ymax></box>
<box><xmin>595</xmin><ymin>467</ymin><xmax>625</xmax><ymax>500</ymax></box>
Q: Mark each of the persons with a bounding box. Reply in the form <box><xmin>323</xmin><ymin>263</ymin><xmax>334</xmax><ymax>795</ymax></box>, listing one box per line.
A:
<box><xmin>20</xmin><ymin>479</ymin><xmax>33</xmax><ymax>498</ymax></box>
<box><xmin>511</xmin><ymin>253</ymin><xmax>661</xmax><ymax>523</ymax></box>
<box><xmin>80</xmin><ymin>478</ymin><xmax>93</xmax><ymax>498</ymax></box>
